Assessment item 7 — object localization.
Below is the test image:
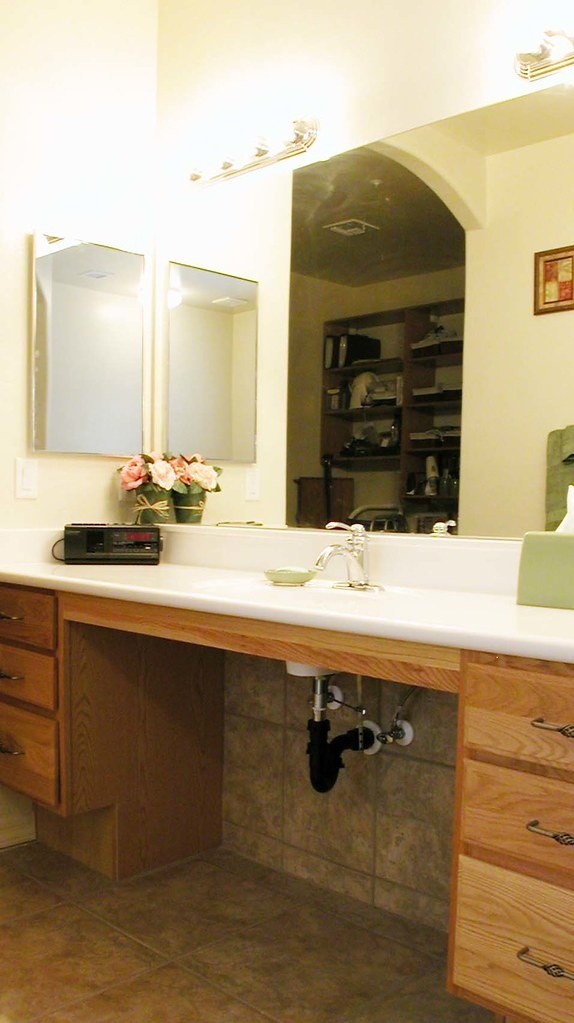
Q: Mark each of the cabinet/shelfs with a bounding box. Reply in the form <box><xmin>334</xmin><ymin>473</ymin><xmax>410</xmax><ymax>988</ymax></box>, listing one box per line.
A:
<box><xmin>445</xmin><ymin>649</ymin><xmax>574</xmax><ymax>1023</ymax></box>
<box><xmin>321</xmin><ymin>298</ymin><xmax>466</xmax><ymax>507</ymax></box>
<box><xmin>0</xmin><ymin>584</ymin><xmax>225</xmax><ymax>879</ymax></box>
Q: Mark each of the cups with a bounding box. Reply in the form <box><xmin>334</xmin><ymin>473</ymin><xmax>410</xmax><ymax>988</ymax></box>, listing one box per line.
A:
<box><xmin>426</xmin><ymin>456</ymin><xmax>439</xmax><ymax>479</ymax></box>
<box><xmin>449</xmin><ymin>479</ymin><xmax>459</xmax><ymax>497</ymax></box>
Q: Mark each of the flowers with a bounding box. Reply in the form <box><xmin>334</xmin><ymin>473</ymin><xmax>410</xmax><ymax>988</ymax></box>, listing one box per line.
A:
<box><xmin>117</xmin><ymin>453</ymin><xmax>176</xmax><ymax>491</ymax></box>
<box><xmin>161</xmin><ymin>451</ymin><xmax>223</xmax><ymax>496</ymax></box>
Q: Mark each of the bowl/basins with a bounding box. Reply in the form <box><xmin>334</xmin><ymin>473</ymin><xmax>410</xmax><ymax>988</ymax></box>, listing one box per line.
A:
<box><xmin>264</xmin><ymin>569</ymin><xmax>317</xmax><ymax>586</ymax></box>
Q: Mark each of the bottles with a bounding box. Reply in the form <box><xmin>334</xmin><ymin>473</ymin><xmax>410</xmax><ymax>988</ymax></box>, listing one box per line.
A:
<box><xmin>438</xmin><ymin>468</ymin><xmax>451</xmax><ymax>496</ymax></box>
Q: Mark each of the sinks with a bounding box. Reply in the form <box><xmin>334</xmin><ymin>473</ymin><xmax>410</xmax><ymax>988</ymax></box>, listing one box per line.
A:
<box><xmin>186</xmin><ymin>572</ymin><xmax>451</xmax><ymax>624</ymax></box>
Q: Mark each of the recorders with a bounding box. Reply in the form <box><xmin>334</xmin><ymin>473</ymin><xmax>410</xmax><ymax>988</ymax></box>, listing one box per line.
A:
<box><xmin>63</xmin><ymin>523</ymin><xmax>163</xmax><ymax>565</ymax></box>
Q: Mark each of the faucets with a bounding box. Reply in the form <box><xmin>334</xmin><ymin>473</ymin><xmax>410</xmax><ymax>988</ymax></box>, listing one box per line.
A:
<box><xmin>313</xmin><ymin>521</ymin><xmax>384</xmax><ymax>593</ymax></box>
<box><xmin>427</xmin><ymin>518</ymin><xmax>457</xmax><ymax>539</ymax></box>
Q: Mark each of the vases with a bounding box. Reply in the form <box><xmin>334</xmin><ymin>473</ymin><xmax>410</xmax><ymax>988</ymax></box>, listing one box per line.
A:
<box><xmin>172</xmin><ymin>488</ymin><xmax>206</xmax><ymax>526</ymax></box>
<box><xmin>135</xmin><ymin>488</ymin><xmax>172</xmax><ymax>524</ymax></box>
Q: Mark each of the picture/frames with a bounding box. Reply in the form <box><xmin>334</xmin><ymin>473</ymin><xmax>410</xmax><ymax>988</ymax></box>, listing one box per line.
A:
<box><xmin>533</xmin><ymin>244</ymin><xmax>574</xmax><ymax>315</ymax></box>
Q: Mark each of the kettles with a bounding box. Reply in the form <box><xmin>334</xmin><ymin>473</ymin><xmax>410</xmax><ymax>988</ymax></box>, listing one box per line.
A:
<box><xmin>348</xmin><ymin>371</ymin><xmax>380</xmax><ymax>408</ymax></box>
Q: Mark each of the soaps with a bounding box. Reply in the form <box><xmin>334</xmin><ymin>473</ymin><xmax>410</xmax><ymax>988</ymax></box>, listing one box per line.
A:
<box><xmin>275</xmin><ymin>567</ymin><xmax>301</xmax><ymax>574</ymax></box>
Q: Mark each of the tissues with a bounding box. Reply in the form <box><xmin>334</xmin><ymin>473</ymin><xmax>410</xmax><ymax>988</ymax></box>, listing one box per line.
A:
<box><xmin>515</xmin><ymin>483</ymin><xmax>574</xmax><ymax>611</ymax></box>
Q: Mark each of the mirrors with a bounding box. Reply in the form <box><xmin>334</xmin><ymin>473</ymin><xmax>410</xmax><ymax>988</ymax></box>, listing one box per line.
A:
<box><xmin>167</xmin><ymin>261</ymin><xmax>260</xmax><ymax>463</ymax></box>
<box><xmin>152</xmin><ymin>82</ymin><xmax>574</xmax><ymax>538</ymax></box>
<box><xmin>29</xmin><ymin>230</ymin><xmax>146</xmax><ymax>460</ymax></box>
